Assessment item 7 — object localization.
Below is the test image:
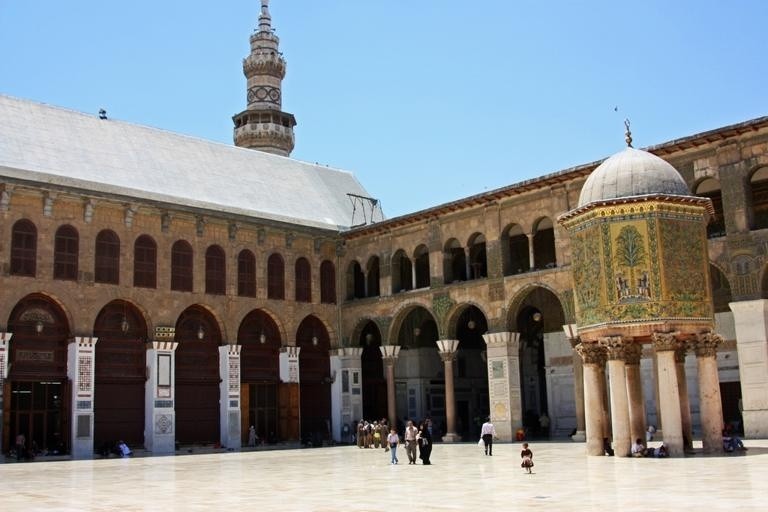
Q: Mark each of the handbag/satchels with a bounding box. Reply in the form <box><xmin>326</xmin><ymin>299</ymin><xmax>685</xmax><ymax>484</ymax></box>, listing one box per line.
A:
<box><xmin>390</xmin><ymin>442</ymin><xmax>396</xmax><ymax>447</ymax></box>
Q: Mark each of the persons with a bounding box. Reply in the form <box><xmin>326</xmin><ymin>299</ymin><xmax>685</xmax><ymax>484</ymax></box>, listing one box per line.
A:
<box><xmin>722</xmin><ymin>429</ymin><xmax>747</xmax><ymax>452</ymax></box>
<box><xmin>683</xmin><ymin>431</ymin><xmax>695</xmax><ymax>453</ymax></box>
<box><xmin>248</xmin><ymin>425</ymin><xmax>258</xmax><ymax>446</ymax></box>
<box><xmin>631</xmin><ymin>437</ymin><xmax>647</xmax><ymax>457</ymax></box>
<box><xmin>538</xmin><ymin>412</ymin><xmax>551</xmax><ymax>439</ymax></box>
<box><xmin>602</xmin><ymin>438</ymin><xmax>615</xmax><ymax>455</ymax></box>
<box><xmin>118</xmin><ymin>439</ymin><xmax>132</xmax><ymax>455</ymax></box>
<box><xmin>646</xmin><ymin>444</ymin><xmax>668</xmax><ymax>457</ymax></box>
<box><xmin>341</xmin><ymin>415</ymin><xmax>433</xmax><ymax>464</ymax></box>
<box><xmin>8</xmin><ymin>432</ymin><xmax>65</xmax><ymax>459</ymax></box>
<box><xmin>481</xmin><ymin>416</ymin><xmax>497</xmax><ymax>456</ymax></box>
<box><xmin>521</xmin><ymin>442</ymin><xmax>533</xmax><ymax>473</ymax></box>
